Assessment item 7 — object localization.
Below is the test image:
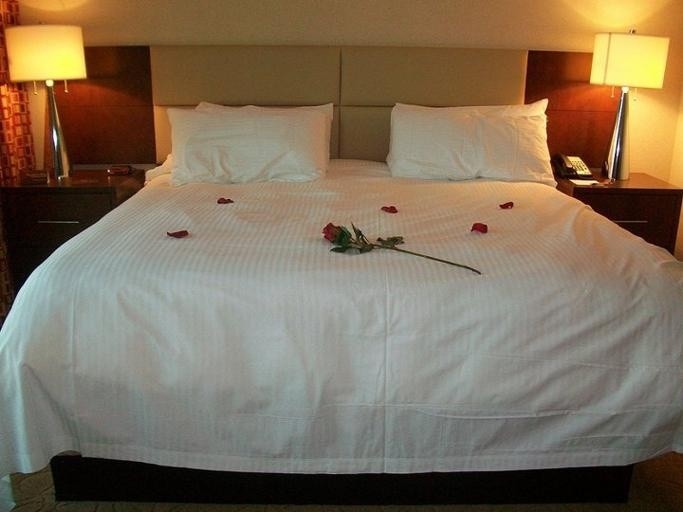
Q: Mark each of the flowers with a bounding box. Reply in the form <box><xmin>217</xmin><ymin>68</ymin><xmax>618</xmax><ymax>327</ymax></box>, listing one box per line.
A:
<box><xmin>323</xmin><ymin>222</ymin><xmax>482</xmax><ymax>275</ymax></box>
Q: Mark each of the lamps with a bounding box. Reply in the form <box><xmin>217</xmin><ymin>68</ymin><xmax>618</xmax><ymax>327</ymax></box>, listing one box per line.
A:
<box><xmin>589</xmin><ymin>29</ymin><xmax>671</xmax><ymax>181</ymax></box>
<box><xmin>3</xmin><ymin>22</ymin><xmax>88</xmax><ymax>178</ymax></box>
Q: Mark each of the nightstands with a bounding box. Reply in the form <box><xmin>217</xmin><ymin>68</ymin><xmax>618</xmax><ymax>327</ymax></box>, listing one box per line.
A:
<box><xmin>556</xmin><ymin>172</ymin><xmax>683</xmax><ymax>255</ymax></box>
<box><xmin>0</xmin><ymin>169</ymin><xmax>145</xmax><ymax>293</ymax></box>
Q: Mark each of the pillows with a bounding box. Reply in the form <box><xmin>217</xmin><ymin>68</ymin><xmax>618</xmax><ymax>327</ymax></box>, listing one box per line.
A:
<box><xmin>385</xmin><ymin>96</ymin><xmax>558</xmax><ymax>188</ymax></box>
<box><xmin>166</xmin><ymin>100</ymin><xmax>333</xmax><ymax>186</ymax></box>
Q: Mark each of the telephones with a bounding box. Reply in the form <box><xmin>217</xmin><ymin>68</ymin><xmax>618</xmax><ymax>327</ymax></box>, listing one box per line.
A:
<box><xmin>550</xmin><ymin>153</ymin><xmax>592</xmax><ymax>178</ymax></box>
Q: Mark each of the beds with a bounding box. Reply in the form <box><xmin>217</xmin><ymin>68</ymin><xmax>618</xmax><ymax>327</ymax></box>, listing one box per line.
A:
<box><xmin>26</xmin><ymin>47</ymin><xmax>683</xmax><ymax>505</ymax></box>
